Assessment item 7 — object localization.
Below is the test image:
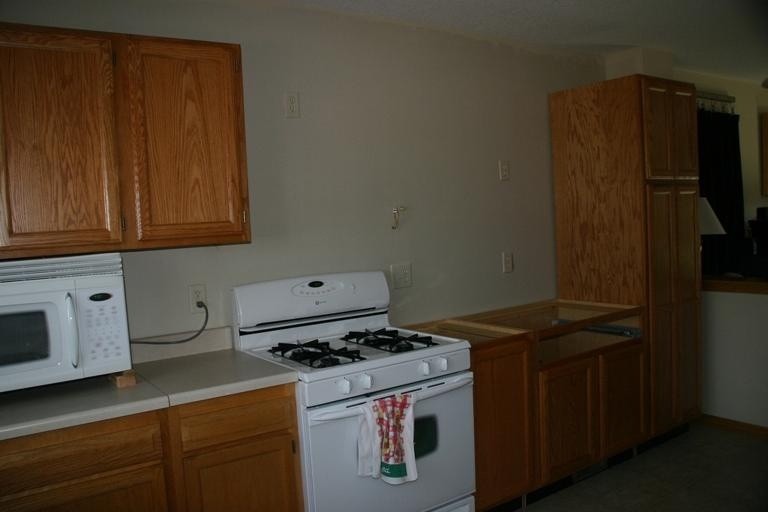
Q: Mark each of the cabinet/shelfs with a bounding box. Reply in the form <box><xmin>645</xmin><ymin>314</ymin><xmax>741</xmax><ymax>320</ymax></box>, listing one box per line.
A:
<box><xmin>550</xmin><ymin>74</ymin><xmax>705</xmax><ymax>436</ymax></box>
<box><xmin>0</xmin><ymin>400</ymin><xmax>168</xmax><ymax>509</ymax></box>
<box><xmin>0</xmin><ymin>25</ymin><xmax>253</xmax><ymax>263</ymax></box>
<box><xmin>536</xmin><ymin>307</ymin><xmax>647</xmax><ymax>496</ymax></box>
<box><xmin>477</xmin><ymin>330</ymin><xmax>536</xmax><ymax>508</ymax></box>
<box><xmin>160</xmin><ymin>383</ymin><xmax>303</xmax><ymax>512</ymax></box>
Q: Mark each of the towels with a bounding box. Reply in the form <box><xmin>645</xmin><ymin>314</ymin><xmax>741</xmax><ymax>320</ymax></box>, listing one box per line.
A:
<box><xmin>353</xmin><ymin>391</ymin><xmax>419</xmax><ymax>484</ymax></box>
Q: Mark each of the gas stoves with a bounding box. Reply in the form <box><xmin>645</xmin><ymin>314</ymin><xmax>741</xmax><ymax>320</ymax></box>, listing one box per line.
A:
<box><xmin>243</xmin><ymin>327</ymin><xmax>471</xmax><ymax>382</ymax></box>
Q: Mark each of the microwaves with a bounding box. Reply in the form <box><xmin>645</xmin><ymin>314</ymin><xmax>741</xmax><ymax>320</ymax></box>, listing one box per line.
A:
<box><xmin>0</xmin><ymin>251</ymin><xmax>133</xmax><ymax>394</ymax></box>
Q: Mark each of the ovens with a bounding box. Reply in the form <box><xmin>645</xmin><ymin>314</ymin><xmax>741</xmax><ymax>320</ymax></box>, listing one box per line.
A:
<box><xmin>303</xmin><ymin>344</ymin><xmax>477</xmax><ymax>512</ymax></box>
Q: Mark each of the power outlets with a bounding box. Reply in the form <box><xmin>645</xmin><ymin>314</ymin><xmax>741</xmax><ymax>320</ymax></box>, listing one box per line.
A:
<box><xmin>188</xmin><ymin>284</ymin><xmax>206</xmax><ymax>314</ymax></box>
